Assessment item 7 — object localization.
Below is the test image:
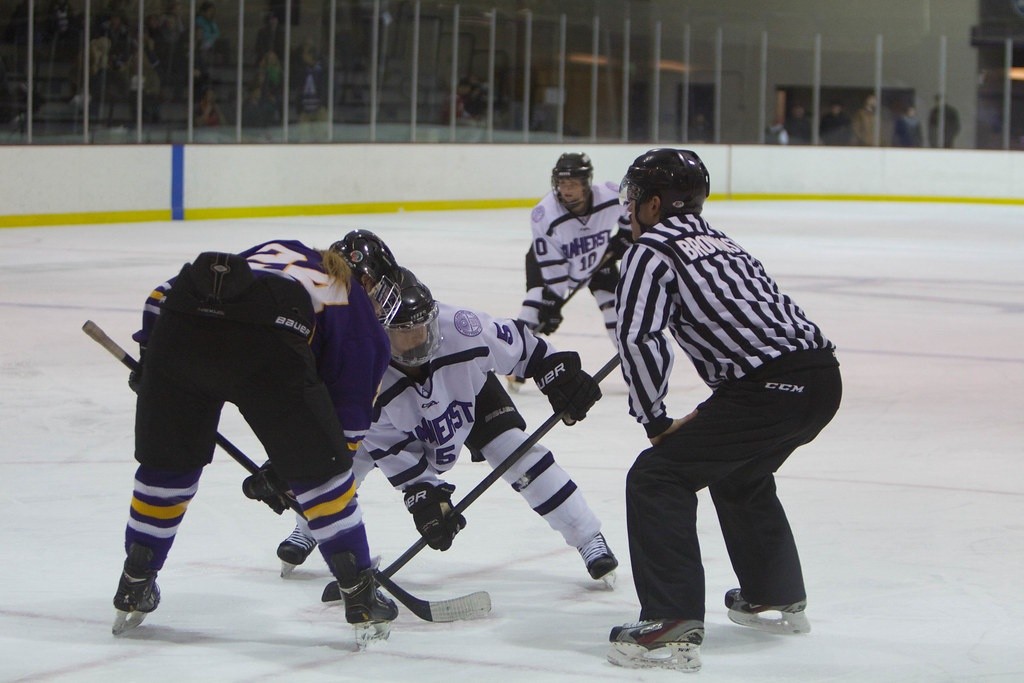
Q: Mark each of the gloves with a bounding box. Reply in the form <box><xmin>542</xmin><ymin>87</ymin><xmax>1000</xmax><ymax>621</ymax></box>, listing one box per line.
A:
<box><xmin>128</xmin><ymin>355</ymin><xmax>145</xmax><ymax>395</ymax></box>
<box><xmin>538</xmin><ymin>286</ymin><xmax>566</xmax><ymax>336</ymax></box>
<box><xmin>531</xmin><ymin>350</ymin><xmax>601</xmax><ymax>426</ymax></box>
<box><xmin>242</xmin><ymin>457</ymin><xmax>297</xmax><ymax>515</ymax></box>
<box><xmin>403</xmin><ymin>481</ymin><xmax>466</xmax><ymax>551</ymax></box>
<box><xmin>604</xmin><ymin>227</ymin><xmax>633</xmax><ymax>260</ymax></box>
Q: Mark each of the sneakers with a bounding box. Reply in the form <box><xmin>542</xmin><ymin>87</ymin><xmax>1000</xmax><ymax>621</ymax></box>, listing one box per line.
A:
<box><xmin>508</xmin><ymin>372</ymin><xmax>524</xmax><ymax>393</ymax></box>
<box><xmin>275</xmin><ymin>521</ymin><xmax>318</xmax><ymax>578</ymax></box>
<box><xmin>331</xmin><ymin>547</ymin><xmax>398</xmax><ymax>651</ymax></box>
<box><xmin>725</xmin><ymin>588</ymin><xmax>811</xmax><ymax>634</ymax></box>
<box><xmin>111</xmin><ymin>541</ymin><xmax>159</xmax><ymax>636</ymax></box>
<box><xmin>606</xmin><ymin>618</ymin><xmax>705</xmax><ymax>673</ymax></box>
<box><xmin>576</xmin><ymin>532</ymin><xmax>619</xmax><ymax>590</ymax></box>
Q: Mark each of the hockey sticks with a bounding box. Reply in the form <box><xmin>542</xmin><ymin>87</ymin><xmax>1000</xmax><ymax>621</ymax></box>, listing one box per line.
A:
<box><xmin>81</xmin><ymin>319</ymin><xmax>494</xmax><ymax>622</ymax></box>
<box><xmin>508</xmin><ymin>249</ymin><xmax>614</xmax><ymax>393</ymax></box>
<box><xmin>320</xmin><ymin>353</ymin><xmax>621</xmax><ymax>604</ymax></box>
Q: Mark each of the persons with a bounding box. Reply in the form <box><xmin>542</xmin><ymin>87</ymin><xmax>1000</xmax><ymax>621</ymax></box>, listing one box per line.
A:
<box><xmin>505</xmin><ymin>151</ymin><xmax>631</xmax><ymax>393</ymax></box>
<box><xmin>275</xmin><ymin>265</ymin><xmax>619</xmax><ymax>594</ymax></box>
<box><xmin>609</xmin><ymin>148</ymin><xmax>842</xmax><ymax>671</ymax></box>
<box><xmin>0</xmin><ymin>0</ymin><xmax>961</xmax><ymax>149</ymax></box>
<box><xmin>112</xmin><ymin>228</ymin><xmax>403</xmax><ymax>651</ymax></box>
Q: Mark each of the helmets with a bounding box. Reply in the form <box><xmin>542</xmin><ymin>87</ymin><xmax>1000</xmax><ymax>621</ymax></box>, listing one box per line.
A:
<box><xmin>378</xmin><ymin>264</ymin><xmax>441</xmax><ymax>368</ymax></box>
<box><xmin>329</xmin><ymin>228</ymin><xmax>396</xmax><ymax>288</ymax></box>
<box><xmin>551</xmin><ymin>151</ymin><xmax>593</xmax><ymax>180</ymax></box>
<box><xmin>617</xmin><ymin>148</ymin><xmax>710</xmax><ymax>222</ymax></box>
